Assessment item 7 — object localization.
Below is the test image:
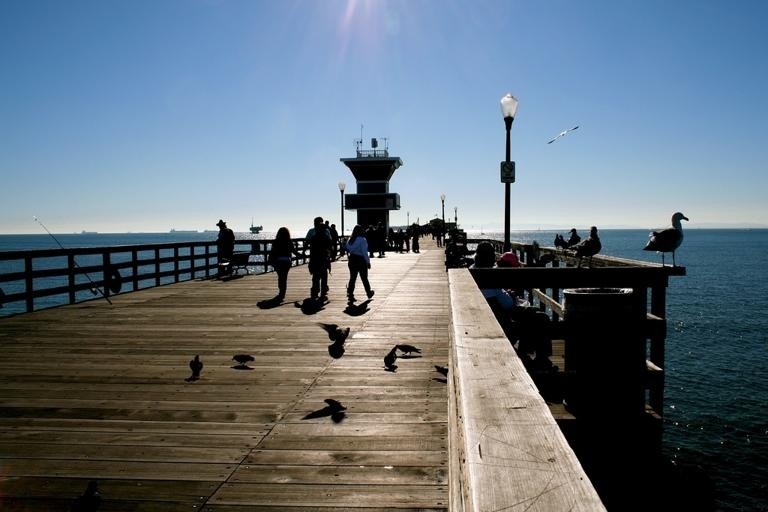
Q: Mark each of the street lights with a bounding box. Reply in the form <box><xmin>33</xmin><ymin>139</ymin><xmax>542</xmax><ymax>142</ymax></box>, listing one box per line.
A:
<box><xmin>338</xmin><ymin>179</ymin><xmax>347</xmax><ymax>236</ymax></box>
<box><xmin>453</xmin><ymin>206</ymin><xmax>458</xmax><ymax>227</ymax></box>
<box><xmin>406</xmin><ymin>210</ymin><xmax>410</xmax><ymax>226</ymax></box>
<box><xmin>440</xmin><ymin>191</ymin><xmax>445</xmax><ymax>221</ymax></box>
<box><xmin>501</xmin><ymin>91</ymin><xmax>518</xmax><ymax>255</ymax></box>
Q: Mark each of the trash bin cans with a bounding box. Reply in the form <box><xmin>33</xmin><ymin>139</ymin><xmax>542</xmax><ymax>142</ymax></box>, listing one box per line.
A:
<box><xmin>560</xmin><ymin>288</ymin><xmax>634</xmax><ymax>411</ymax></box>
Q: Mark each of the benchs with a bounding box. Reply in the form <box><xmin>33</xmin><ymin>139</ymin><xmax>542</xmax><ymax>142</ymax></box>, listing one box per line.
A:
<box><xmin>218</xmin><ymin>251</ymin><xmax>250</xmax><ymax>276</ymax></box>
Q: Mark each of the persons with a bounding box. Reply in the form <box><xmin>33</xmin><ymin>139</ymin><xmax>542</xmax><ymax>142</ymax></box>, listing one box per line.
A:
<box><xmin>493</xmin><ymin>251</ymin><xmax>532</xmax><ymax>307</ymax></box>
<box><xmin>212</xmin><ymin>220</ymin><xmax>235</xmax><ymax>277</ymax></box>
<box><xmin>363</xmin><ymin>221</ymin><xmax>444</xmax><ymax>259</ymax></box>
<box><xmin>266</xmin><ymin>227</ymin><xmax>301</xmax><ymax>300</ymax></box>
<box><xmin>301</xmin><ymin>215</ymin><xmax>349</xmax><ymax>302</ymax></box>
<box><xmin>466</xmin><ymin>241</ymin><xmax>559</xmax><ymax>374</ymax></box>
<box><xmin>342</xmin><ymin>224</ymin><xmax>374</xmax><ymax>298</ymax></box>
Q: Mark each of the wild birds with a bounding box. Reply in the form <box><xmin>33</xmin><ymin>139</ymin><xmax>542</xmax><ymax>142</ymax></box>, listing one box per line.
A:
<box><xmin>188</xmin><ymin>354</ymin><xmax>203</xmax><ymax>378</ymax></box>
<box><xmin>434</xmin><ymin>363</ymin><xmax>449</xmax><ymax>377</ymax></box>
<box><xmin>384</xmin><ymin>346</ymin><xmax>399</xmax><ymax>370</ymax></box>
<box><xmin>554</xmin><ymin>228</ymin><xmax>580</xmax><ymax>255</ymax></box>
<box><xmin>397</xmin><ymin>343</ymin><xmax>421</xmax><ymax>356</ymax></box>
<box><xmin>643</xmin><ymin>211</ymin><xmax>690</xmax><ymax>267</ymax></box>
<box><xmin>571</xmin><ymin>225</ymin><xmax>601</xmax><ymax>269</ymax></box>
<box><xmin>324</xmin><ymin>399</ymin><xmax>347</xmax><ymax>412</ymax></box>
<box><xmin>547</xmin><ymin>125</ymin><xmax>580</xmax><ymax>144</ymax></box>
<box><xmin>317</xmin><ymin>320</ymin><xmax>351</xmax><ymax>345</ymax></box>
<box><xmin>231</xmin><ymin>354</ymin><xmax>256</xmax><ymax>366</ymax></box>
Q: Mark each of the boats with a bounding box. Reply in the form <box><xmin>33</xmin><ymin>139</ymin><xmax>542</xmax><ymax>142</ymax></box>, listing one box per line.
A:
<box><xmin>250</xmin><ymin>225</ymin><xmax>264</xmax><ymax>234</ymax></box>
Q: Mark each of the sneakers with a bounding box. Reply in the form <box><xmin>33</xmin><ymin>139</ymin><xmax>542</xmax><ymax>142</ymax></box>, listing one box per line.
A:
<box><xmin>311</xmin><ymin>295</ymin><xmax>328</xmax><ymax>301</ymax></box>
<box><xmin>533</xmin><ymin>357</ymin><xmax>560</xmax><ymax>371</ymax></box>
<box><xmin>367</xmin><ymin>290</ymin><xmax>374</xmax><ymax>299</ymax></box>
